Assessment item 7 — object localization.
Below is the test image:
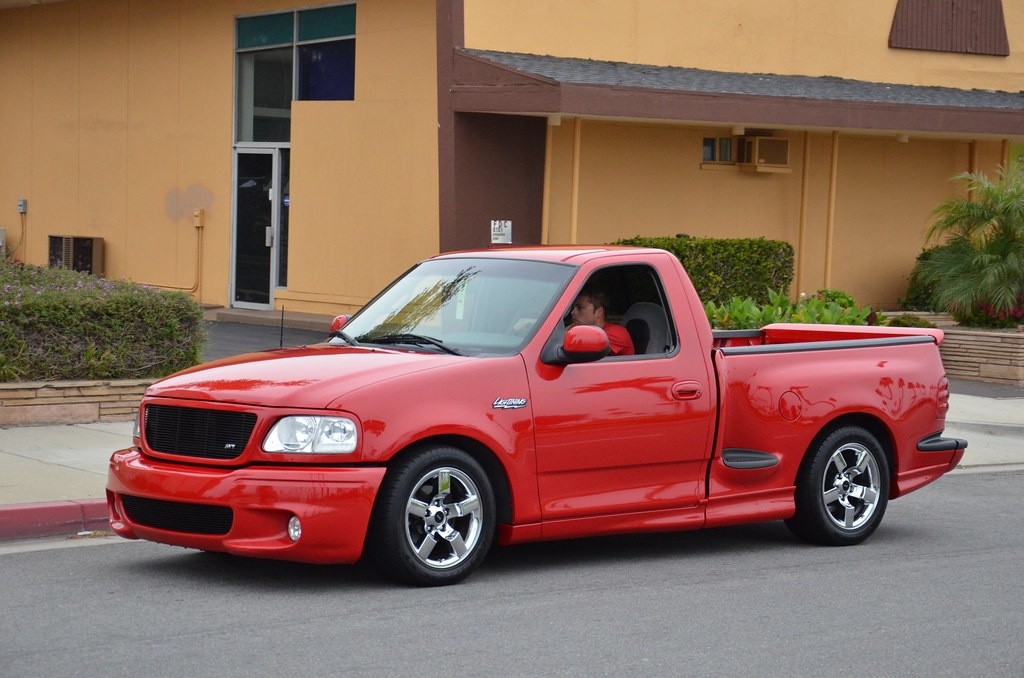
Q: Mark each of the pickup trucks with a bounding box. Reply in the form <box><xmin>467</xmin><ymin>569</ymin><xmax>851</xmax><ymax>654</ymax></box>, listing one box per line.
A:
<box><xmin>104</xmin><ymin>242</ymin><xmax>970</xmax><ymax>589</ymax></box>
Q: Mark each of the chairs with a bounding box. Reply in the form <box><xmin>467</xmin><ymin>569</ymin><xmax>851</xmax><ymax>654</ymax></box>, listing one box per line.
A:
<box><xmin>621</xmin><ymin>302</ymin><xmax>668</xmax><ymax>354</ymax></box>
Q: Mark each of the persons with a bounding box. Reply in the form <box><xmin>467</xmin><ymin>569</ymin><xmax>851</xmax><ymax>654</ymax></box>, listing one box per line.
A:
<box><xmin>513</xmin><ymin>284</ymin><xmax>636</xmax><ymax>355</ymax></box>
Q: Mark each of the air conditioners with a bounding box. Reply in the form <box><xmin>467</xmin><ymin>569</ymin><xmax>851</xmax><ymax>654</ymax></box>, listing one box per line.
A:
<box><xmin>738</xmin><ymin>136</ymin><xmax>789</xmax><ymax>166</ymax></box>
